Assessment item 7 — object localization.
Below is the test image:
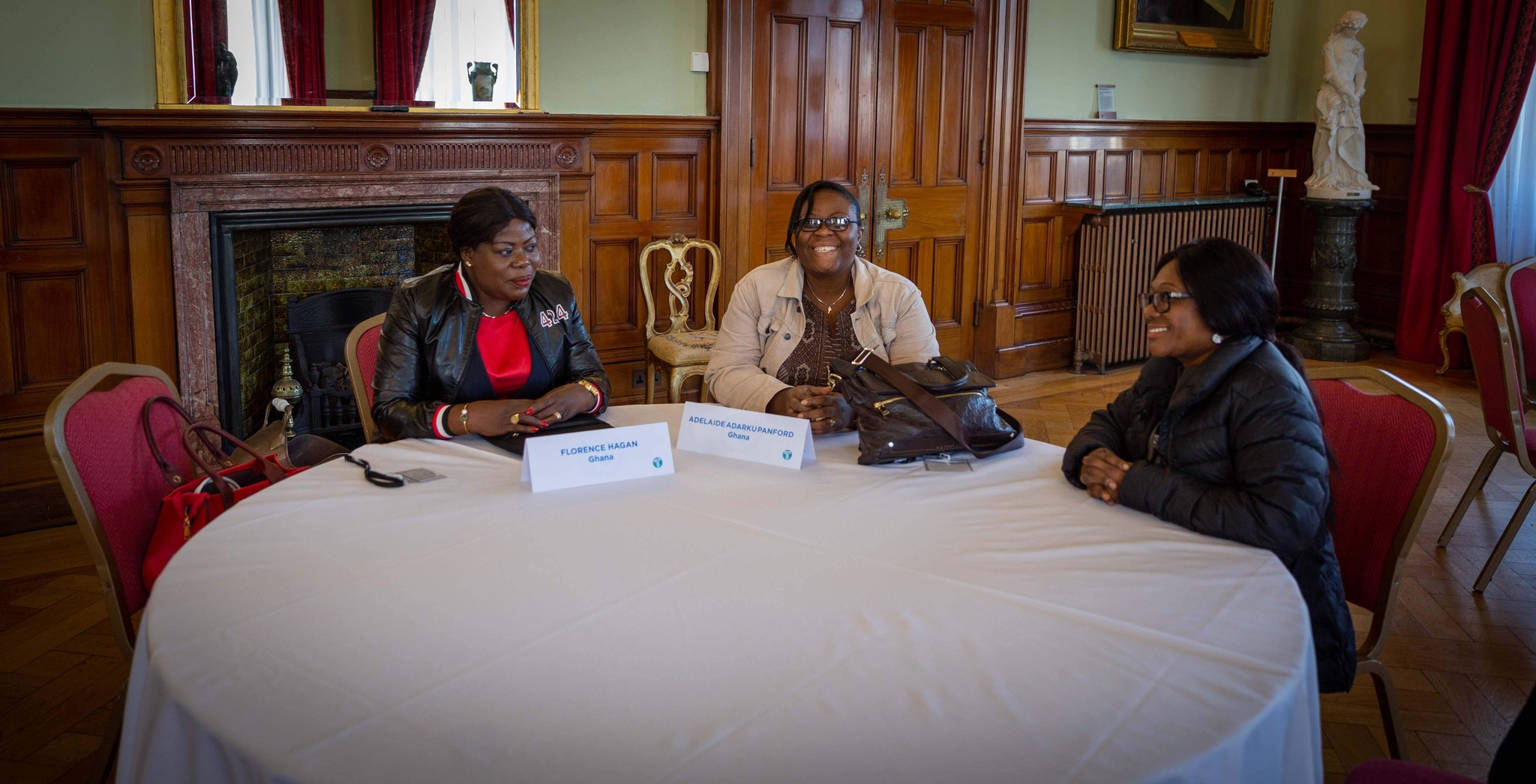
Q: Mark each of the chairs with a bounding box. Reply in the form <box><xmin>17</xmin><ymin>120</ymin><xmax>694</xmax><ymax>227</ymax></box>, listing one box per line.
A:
<box><xmin>1438</xmin><ymin>262</ymin><xmax>1509</xmax><ymax>374</ymax></box>
<box><xmin>640</xmin><ymin>232</ymin><xmax>721</xmax><ymax>404</ymax></box>
<box><xmin>1500</xmin><ymin>256</ymin><xmax>1536</xmax><ymax>414</ymax></box>
<box><xmin>287</xmin><ymin>286</ymin><xmax>401</xmax><ymax>445</ymax></box>
<box><xmin>1437</xmin><ymin>287</ymin><xmax>1536</xmax><ymax>593</ymax></box>
<box><xmin>345</xmin><ymin>311</ymin><xmax>387</xmax><ymax>444</ymax></box>
<box><xmin>1304</xmin><ymin>368</ymin><xmax>1456</xmax><ymax>759</ymax></box>
<box><xmin>45</xmin><ymin>363</ymin><xmax>199</xmax><ymax>784</ymax></box>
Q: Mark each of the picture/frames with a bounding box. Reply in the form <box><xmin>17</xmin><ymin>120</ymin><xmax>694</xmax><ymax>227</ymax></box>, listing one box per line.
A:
<box><xmin>1113</xmin><ymin>0</ymin><xmax>1273</xmax><ymax>58</ymax></box>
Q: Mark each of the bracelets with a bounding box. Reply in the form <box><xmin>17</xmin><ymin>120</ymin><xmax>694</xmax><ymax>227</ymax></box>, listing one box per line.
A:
<box><xmin>460</xmin><ymin>403</ymin><xmax>470</xmax><ymax>435</ymax></box>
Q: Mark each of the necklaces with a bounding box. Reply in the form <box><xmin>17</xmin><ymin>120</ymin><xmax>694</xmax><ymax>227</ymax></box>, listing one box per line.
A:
<box><xmin>805</xmin><ymin>273</ymin><xmax>851</xmax><ymax>314</ymax></box>
<box><xmin>482</xmin><ymin>306</ymin><xmax>513</xmax><ymax>319</ymax></box>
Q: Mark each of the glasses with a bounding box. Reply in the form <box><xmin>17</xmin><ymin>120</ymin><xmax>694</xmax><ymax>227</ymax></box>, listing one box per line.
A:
<box><xmin>796</xmin><ymin>217</ymin><xmax>858</xmax><ymax>231</ymax></box>
<box><xmin>1137</xmin><ymin>290</ymin><xmax>1191</xmax><ymax>313</ymax></box>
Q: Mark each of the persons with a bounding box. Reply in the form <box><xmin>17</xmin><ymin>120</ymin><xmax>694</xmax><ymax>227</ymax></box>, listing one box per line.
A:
<box><xmin>704</xmin><ymin>180</ymin><xmax>940</xmax><ymax>435</ymax></box>
<box><xmin>1303</xmin><ymin>10</ymin><xmax>1380</xmax><ymax>191</ymax></box>
<box><xmin>1060</xmin><ymin>237</ymin><xmax>1358</xmax><ymax>694</ymax></box>
<box><xmin>371</xmin><ymin>185</ymin><xmax>611</xmax><ymax>441</ymax></box>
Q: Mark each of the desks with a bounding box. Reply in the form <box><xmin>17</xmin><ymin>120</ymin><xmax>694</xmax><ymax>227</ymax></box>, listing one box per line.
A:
<box><xmin>1271</xmin><ymin>197</ymin><xmax>1405</xmax><ymax>360</ymax></box>
<box><xmin>116</xmin><ymin>404</ymin><xmax>1331</xmax><ymax>784</ymax></box>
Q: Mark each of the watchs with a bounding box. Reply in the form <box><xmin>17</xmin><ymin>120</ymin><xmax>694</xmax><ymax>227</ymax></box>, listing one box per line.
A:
<box><xmin>578</xmin><ymin>380</ymin><xmax>599</xmax><ymax>400</ymax></box>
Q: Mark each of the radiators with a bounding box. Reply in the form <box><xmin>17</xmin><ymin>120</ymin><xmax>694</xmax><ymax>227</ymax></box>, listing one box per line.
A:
<box><xmin>1071</xmin><ymin>206</ymin><xmax>1265</xmax><ymax>375</ymax></box>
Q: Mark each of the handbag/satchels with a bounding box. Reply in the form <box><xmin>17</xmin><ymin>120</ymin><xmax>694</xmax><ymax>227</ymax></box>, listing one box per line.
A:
<box><xmin>826</xmin><ymin>347</ymin><xmax>1024</xmax><ymax>466</ymax></box>
<box><xmin>142</xmin><ymin>395</ymin><xmax>311</xmax><ymax>591</ymax></box>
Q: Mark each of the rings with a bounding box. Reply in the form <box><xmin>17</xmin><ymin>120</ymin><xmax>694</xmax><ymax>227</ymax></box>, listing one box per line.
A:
<box><xmin>511</xmin><ymin>414</ymin><xmax>519</xmax><ymax>425</ymax></box>
<box><xmin>828</xmin><ymin>418</ymin><xmax>836</xmax><ymax>426</ymax></box>
<box><xmin>554</xmin><ymin>412</ymin><xmax>562</xmax><ymax>420</ymax></box>
